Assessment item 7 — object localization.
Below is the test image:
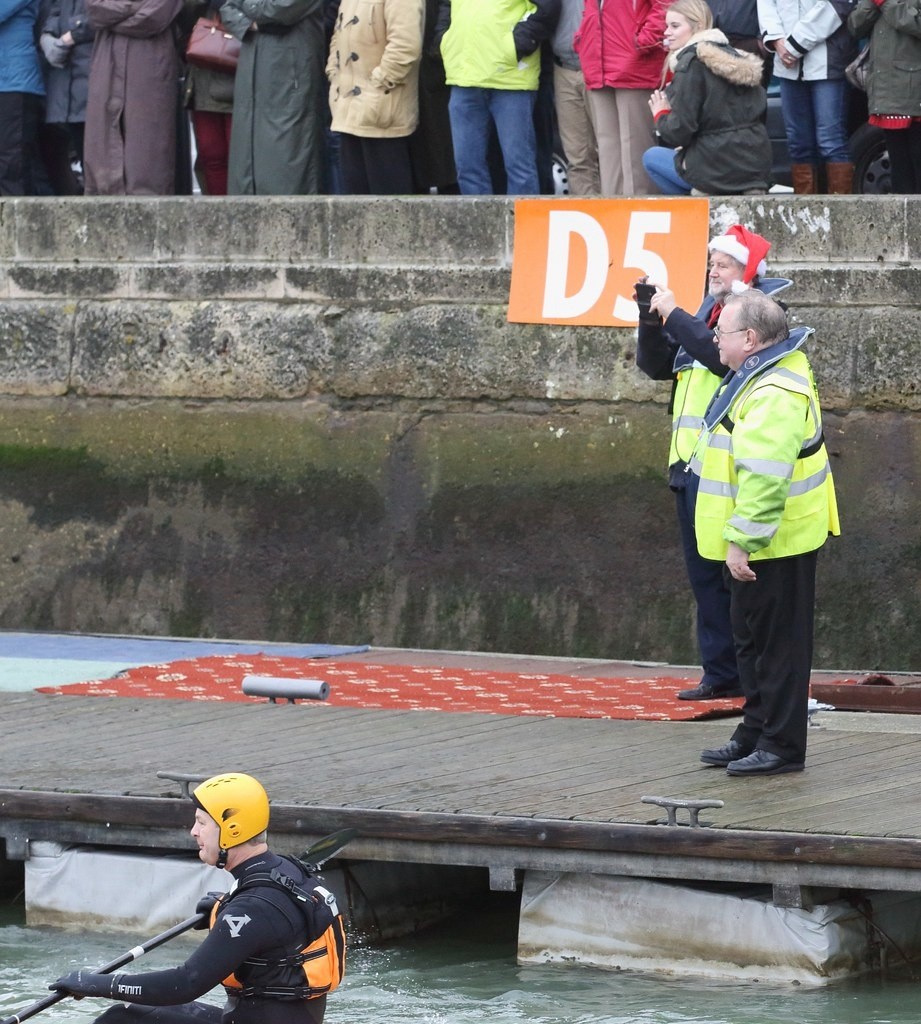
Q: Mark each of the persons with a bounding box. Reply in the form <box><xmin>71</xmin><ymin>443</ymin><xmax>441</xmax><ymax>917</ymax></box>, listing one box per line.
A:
<box><xmin>642</xmin><ymin>0</ymin><xmax>772</xmax><ymax>196</ymax></box>
<box><xmin>633</xmin><ymin>226</ymin><xmax>772</xmax><ymax>701</ymax></box>
<box><xmin>695</xmin><ymin>293</ymin><xmax>829</xmax><ymax>777</ymax></box>
<box><xmin>48</xmin><ymin>773</ymin><xmax>346</xmax><ymax>1024</ymax></box>
<box><xmin>0</xmin><ymin>0</ymin><xmax>921</xmax><ymax>195</ymax></box>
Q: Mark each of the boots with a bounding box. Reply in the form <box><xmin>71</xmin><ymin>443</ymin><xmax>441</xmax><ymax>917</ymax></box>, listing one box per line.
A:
<box><xmin>792</xmin><ymin>162</ymin><xmax>816</xmax><ymax>194</ymax></box>
<box><xmin>826</xmin><ymin>162</ymin><xmax>855</xmax><ymax>194</ymax></box>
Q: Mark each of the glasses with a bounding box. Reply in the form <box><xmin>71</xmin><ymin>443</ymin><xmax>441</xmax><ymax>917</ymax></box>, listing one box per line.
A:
<box><xmin>714</xmin><ymin>326</ymin><xmax>747</xmax><ymax>337</ymax></box>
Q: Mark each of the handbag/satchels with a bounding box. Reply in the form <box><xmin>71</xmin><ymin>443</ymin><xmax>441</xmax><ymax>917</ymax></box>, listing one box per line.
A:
<box><xmin>845</xmin><ymin>44</ymin><xmax>871</xmax><ymax>92</ymax></box>
<box><xmin>187</xmin><ymin>17</ymin><xmax>242</xmax><ymax>66</ymax></box>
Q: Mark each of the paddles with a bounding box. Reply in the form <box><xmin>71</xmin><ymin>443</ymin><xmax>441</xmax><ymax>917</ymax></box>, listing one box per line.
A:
<box><xmin>1</xmin><ymin>825</ymin><xmax>357</xmax><ymax>1024</ymax></box>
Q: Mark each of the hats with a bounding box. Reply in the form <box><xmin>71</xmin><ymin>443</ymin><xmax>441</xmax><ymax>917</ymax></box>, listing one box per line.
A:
<box><xmin>709</xmin><ymin>224</ymin><xmax>771</xmax><ymax>293</ymax></box>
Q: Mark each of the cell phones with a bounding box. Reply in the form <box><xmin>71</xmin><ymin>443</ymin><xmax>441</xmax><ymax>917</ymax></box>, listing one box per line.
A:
<box><xmin>635</xmin><ymin>284</ymin><xmax>657</xmax><ymax>304</ymax></box>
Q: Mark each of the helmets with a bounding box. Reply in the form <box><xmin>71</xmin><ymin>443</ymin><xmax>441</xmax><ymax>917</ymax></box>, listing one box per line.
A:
<box><xmin>192</xmin><ymin>772</ymin><xmax>271</xmax><ymax>849</ymax></box>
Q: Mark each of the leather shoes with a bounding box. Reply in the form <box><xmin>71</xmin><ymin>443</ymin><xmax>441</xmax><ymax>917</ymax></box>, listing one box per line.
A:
<box><xmin>678</xmin><ymin>682</ymin><xmax>743</xmax><ymax>698</ymax></box>
<box><xmin>726</xmin><ymin>749</ymin><xmax>804</xmax><ymax>777</ymax></box>
<box><xmin>700</xmin><ymin>740</ymin><xmax>755</xmax><ymax>766</ymax></box>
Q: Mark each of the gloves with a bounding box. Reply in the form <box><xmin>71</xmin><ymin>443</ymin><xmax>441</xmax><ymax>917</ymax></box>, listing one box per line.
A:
<box><xmin>194</xmin><ymin>891</ymin><xmax>227</xmax><ymax>929</ymax></box>
<box><xmin>41</xmin><ymin>34</ymin><xmax>69</xmax><ymax>69</ymax></box>
<box><xmin>47</xmin><ymin>970</ymin><xmax>126</xmax><ymax>1001</ymax></box>
